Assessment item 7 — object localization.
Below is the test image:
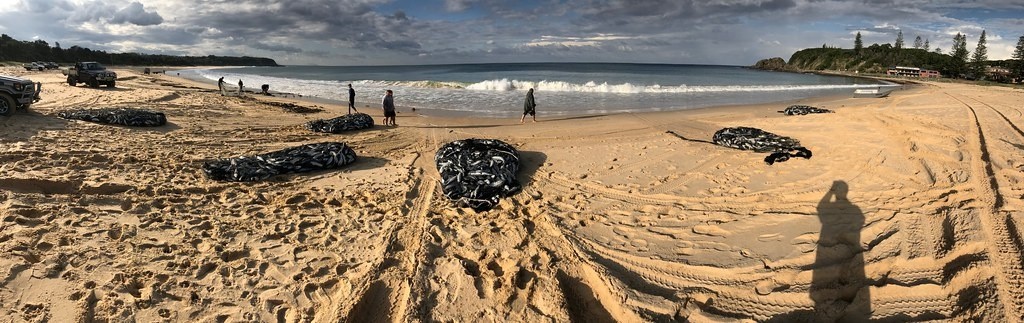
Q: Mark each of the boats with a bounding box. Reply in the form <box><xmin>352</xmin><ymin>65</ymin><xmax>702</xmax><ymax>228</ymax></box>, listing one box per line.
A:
<box><xmin>853</xmin><ymin>89</ymin><xmax>893</xmax><ymax>98</ymax></box>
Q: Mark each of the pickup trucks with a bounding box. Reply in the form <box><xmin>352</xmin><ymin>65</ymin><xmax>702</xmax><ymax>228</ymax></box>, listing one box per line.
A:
<box><xmin>23</xmin><ymin>62</ymin><xmax>44</xmax><ymax>70</ymax></box>
<box><xmin>64</xmin><ymin>62</ymin><xmax>116</xmax><ymax>88</ymax></box>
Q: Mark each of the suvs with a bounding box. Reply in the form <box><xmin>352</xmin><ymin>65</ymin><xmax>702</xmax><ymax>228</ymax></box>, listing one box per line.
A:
<box><xmin>0</xmin><ymin>75</ymin><xmax>41</xmax><ymax>116</ymax></box>
<box><xmin>38</xmin><ymin>62</ymin><xmax>58</xmax><ymax>68</ymax></box>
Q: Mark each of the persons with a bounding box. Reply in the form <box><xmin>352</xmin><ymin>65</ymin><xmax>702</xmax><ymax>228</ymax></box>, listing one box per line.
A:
<box><xmin>218</xmin><ymin>77</ymin><xmax>226</xmax><ymax>91</ymax></box>
<box><xmin>348</xmin><ymin>84</ymin><xmax>357</xmax><ymax>114</ymax></box>
<box><xmin>520</xmin><ymin>88</ymin><xmax>537</xmax><ymax>123</ymax></box>
<box><xmin>383</xmin><ymin>90</ymin><xmax>397</xmax><ymax>127</ymax></box>
<box><xmin>238</xmin><ymin>79</ymin><xmax>244</xmax><ymax>94</ymax></box>
<box><xmin>381</xmin><ymin>91</ymin><xmax>394</xmax><ymax>124</ymax></box>
